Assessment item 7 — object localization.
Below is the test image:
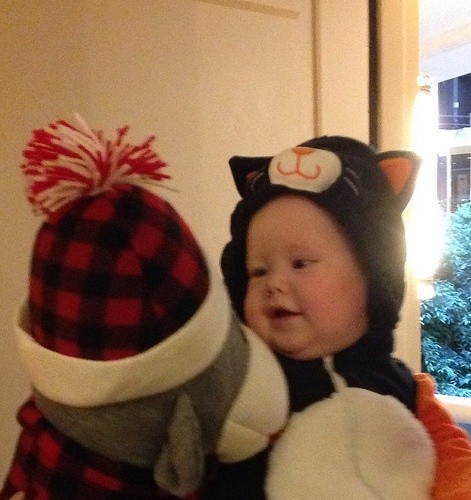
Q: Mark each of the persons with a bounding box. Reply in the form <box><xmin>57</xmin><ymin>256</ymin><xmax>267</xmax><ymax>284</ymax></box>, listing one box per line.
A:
<box><xmin>220</xmin><ymin>136</ymin><xmax>471</xmax><ymax>500</ymax></box>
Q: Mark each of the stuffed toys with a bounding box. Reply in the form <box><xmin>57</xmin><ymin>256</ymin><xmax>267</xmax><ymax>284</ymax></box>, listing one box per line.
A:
<box><xmin>1</xmin><ymin>109</ymin><xmax>292</xmax><ymax>500</ymax></box>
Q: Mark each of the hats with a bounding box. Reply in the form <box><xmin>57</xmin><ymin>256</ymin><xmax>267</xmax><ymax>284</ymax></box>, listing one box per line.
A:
<box><xmin>220</xmin><ymin>135</ymin><xmax>422</xmax><ymax>358</ymax></box>
